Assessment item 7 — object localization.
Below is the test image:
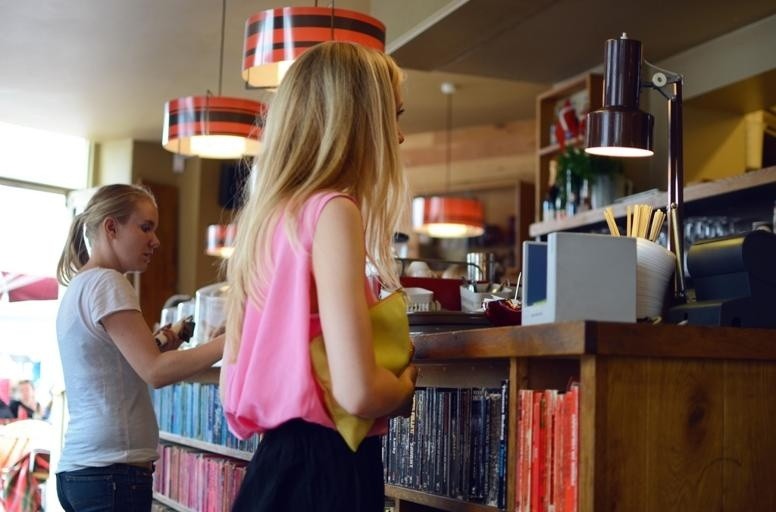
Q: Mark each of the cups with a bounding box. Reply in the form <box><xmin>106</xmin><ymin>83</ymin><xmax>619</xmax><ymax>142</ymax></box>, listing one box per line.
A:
<box><xmin>681</xmin><ymin>219</ymin><xmax>736</xmax><ymax>244</ymax></box>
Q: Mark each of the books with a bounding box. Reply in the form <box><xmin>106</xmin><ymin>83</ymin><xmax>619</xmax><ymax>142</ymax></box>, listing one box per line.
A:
<box><xmin>153</xmin><ymin>380</ymin><xmax>262</xmax><ymax>512</ymax></box>
<box><xmin>382</xmin><ymin>379</ymin><xmax>510</xmax><ymax>511</ymax></box>
<box><xmin>516</xmin><ymin>383</ymin><xmax>579</xmax><ymax>511</ymax></box>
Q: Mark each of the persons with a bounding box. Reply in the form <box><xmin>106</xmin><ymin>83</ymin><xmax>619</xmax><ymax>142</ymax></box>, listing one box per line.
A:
<box><xmin>216</xmin><ymin>41</ymin><xmax>421</xmax><ymax>510</ymax></box>
<box><xmin>54</xmin><ymin>182</ymin><xmax>225</xmax><ymax>512</ymax></box>
<box><xmin>8</xmin><ymin>379</ymin><xmax>42</xmax><ymax>420</ymax></box>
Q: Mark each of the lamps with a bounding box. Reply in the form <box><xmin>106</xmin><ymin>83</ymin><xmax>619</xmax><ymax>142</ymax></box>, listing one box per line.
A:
<box><xmin>410</xmin><ymin>82</ymin><xmax>487</xmax><ymax>241</ymax></box>
<box><xmin>160</xmin><ymin>0</ymin><xmax>392</xmax><ymax>159</ymax></box>
<box><xmin>583</xmin><ymin>29</ymin><xmax>690</xmax><ymax>325</ymax></box>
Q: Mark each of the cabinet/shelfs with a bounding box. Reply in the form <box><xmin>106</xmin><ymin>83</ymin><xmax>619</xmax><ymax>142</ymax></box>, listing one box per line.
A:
<box><xmin>153</xmin><ymin>318</ymin><xmax>776</xmax><ymax>512</ymax></box>
<box><xmin>404</xmin><ymin>182</ymin><xmax>535</xmax><ymax>288</ymax></box>
<box><xmin>529</xmin><ymin>69</ymin><xmax>776</xmax><ymax>243</ymax></box>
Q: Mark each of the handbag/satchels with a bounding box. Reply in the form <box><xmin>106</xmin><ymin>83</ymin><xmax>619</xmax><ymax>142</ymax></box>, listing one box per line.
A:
<box><xmin>309</xmin><ymin>285</ymin><xmax>417</xmax><ymax>455</ymax></box>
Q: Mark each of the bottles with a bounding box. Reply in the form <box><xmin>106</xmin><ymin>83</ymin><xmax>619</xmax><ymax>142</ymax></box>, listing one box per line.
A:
<box><xmin>394</xmin><ymin>230</ymin><xmax>410</xmax><ymax>258</ymax></box>
<box><xmin>465</xmin><ymin>249</ymin><xmax>497</xmax><ymax>282</ymax></box>
